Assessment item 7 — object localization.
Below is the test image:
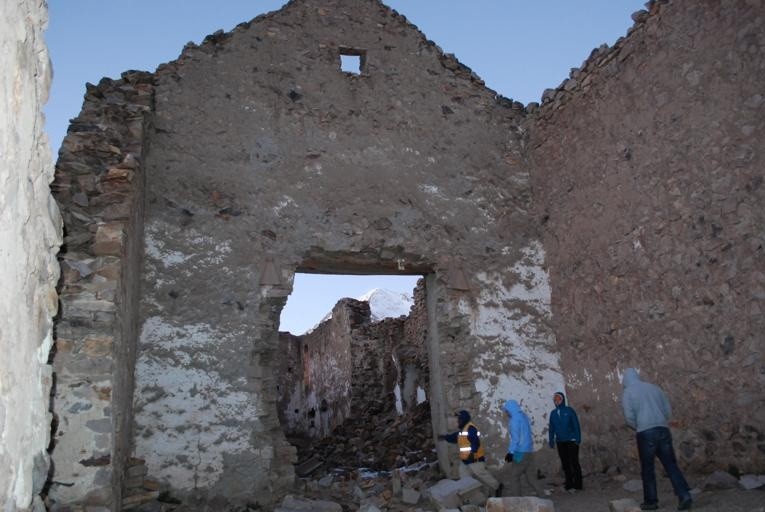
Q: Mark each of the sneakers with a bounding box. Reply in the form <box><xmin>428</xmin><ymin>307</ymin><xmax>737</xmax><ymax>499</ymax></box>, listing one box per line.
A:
<box><xmin>640</xmin><ymin>502</ymin><xmax>658</xmax><ymax>510</ymax></box>
<box><xmin>564</xmin><ymin>484</ymin><xmax>583</xmax><ymax>490</ymax></box>
<box><xmin>678</xmin><ymin>498</ymin><xmax>693</xmax><ymax>509</ymax></box>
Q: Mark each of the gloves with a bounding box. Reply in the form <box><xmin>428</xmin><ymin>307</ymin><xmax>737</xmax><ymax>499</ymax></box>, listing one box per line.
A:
<box><xmin>437</xmin><ymin>435</ymin><xmax>447</xmax><ymax>441</ymax></box>
<box><xmin>505</xmin><ymin>452</ymin><xmax>513</xmax><ymax>463</ymax></box>
<box><xmin>469</xmin><ymin>452</ymin><xmax>478</xmax><ymax>463</ymax></box>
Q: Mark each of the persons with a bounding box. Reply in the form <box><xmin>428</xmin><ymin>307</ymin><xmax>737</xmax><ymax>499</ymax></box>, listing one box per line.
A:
<box><xmin>437</xmin><ymin>410</ymin><xmax>504</xmax><ymax>498</ymax></box>
<box><xmin>549</xmin><ymin>392</ymin><xmax>584</xmax><ymax>491</ymax></box>
<box><xmin>621</xmin><ymin>368</ymin><xmax>692</xmax><ymax>510</ymax></box>
<box><xmin>502</xmin><ymin>399</ymin><xmax>546</xmax><ymax>499</ymax></box>
